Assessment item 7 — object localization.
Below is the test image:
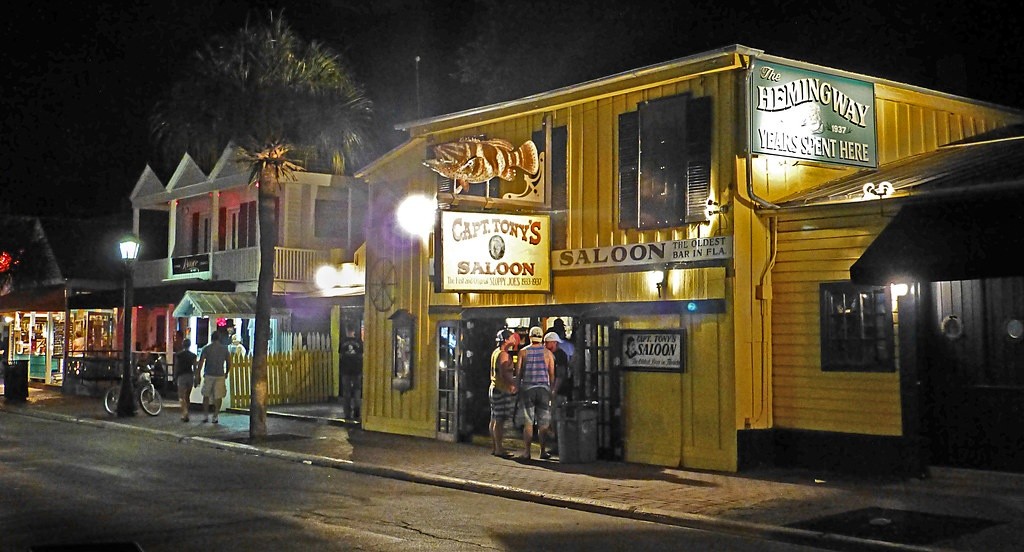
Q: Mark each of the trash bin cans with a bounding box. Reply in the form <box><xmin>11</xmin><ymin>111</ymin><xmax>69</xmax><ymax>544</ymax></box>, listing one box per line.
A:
<box><xmin>554</xmin><ymin>399</ymin><xmax>600</xmax><ymax>465</ymax></box>
<box><xmin>3</xmin><ymin>358</ymin><xmax>30</xmax><ymax>402</ymax></box>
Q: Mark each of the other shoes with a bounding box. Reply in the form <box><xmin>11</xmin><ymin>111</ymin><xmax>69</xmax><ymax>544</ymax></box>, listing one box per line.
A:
<box><xmin>201</xmin><ymin>419</ymin><xmax>207</xmax><ymax>422</ymax></box>
<box><xmin>180</xmin><ymin>416</ymin><xmax>189</xmax><ymax>421</ymax></box>
<box><xmin>212</xmin><ymin>420</ymin><xmax>218</xmax><ymax>423</ymax></box>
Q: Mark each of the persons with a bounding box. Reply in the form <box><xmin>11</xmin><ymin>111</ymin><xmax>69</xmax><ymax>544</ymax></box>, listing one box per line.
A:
<box><xmin>231</xmin><ymin>334</ymin><xmax>246</xmax><ymax>359</ymax></box>
<box><xmin>195</xmin><ymin>330</ymin><xmax>232</xmax><ymax>423</ymax></box>
<box><xmin>172</xmin><ymin>338</ymin><xmax>197</xmax><ymax>421</ymax></box>
<box><xmin>226</xmin><ymin>326</ymin><xmax>235</xmax><ymax>344</ymax></box>
<box><xmin>488</xmin><ymin>318</ymin><xmax>576</xmax><ymax>459</ymax></box>
<box><xmin>336</xmin><ymin>322</ymin><xmax>364</xmax><ymax>420</ymax></box>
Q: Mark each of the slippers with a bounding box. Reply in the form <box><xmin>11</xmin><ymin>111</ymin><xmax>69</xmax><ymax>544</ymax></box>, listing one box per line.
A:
<box><xmin>491</xmin><ymin>450</ymin><xmax>513</xmax><ymax>456</ymax></box>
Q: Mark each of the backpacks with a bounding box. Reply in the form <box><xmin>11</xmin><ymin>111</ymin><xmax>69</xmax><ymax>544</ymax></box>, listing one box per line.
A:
<box><xmin>340</xmin><ymin>340</ymin><xmax>363</xmax><ymax>376</ymax></box>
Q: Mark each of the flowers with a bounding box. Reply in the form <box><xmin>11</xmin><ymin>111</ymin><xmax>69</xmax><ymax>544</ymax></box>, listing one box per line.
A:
<box><xmin>217</xmin><ymin>318</ymin><xmax>227</xmax><ymax>326</ymax></box>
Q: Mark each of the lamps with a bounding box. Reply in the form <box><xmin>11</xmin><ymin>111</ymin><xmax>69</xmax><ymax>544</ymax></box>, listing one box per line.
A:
<box><xmin>186</xmin><ymin>327</ymin><xmax>192</xmax><ymax>335</ymax></box>
<box><xmin>653</xmin><ymin>267</ymin><xmax>666</xmax><ymax>299</ymax></box>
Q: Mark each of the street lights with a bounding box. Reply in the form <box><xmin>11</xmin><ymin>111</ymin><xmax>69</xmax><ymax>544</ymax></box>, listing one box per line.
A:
<box><xmin>117</xmin><ymin>234</ymin><xmax>141</xmax><ymax>417</ymax></box>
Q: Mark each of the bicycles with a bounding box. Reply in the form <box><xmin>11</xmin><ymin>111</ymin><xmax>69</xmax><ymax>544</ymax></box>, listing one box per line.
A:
<box><xmin>103</xmin><ymin>356</ymin><xmax>163</xmax><ymax>416</ymax></box>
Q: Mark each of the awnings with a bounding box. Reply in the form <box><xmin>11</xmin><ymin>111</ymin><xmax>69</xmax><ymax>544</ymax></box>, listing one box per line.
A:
<box><xmin>849</xmin><ymin>195</ymin><xmax>1024</xmax><ymax>284</ymax></box>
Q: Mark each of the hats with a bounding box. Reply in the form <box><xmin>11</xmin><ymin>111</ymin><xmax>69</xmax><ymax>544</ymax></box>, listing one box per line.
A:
<box><xmin>544</xmin><ymin>332</ymin><xmax>564</xmax><ymax>343</ymax></box>
<box><xmin>528</xmin><ymin>327</ymin><xmax>543</xmax><ymax>342</ymax></box>
<box><xmin>496</xmin><ymin>329</ymin><xmax>513</xmax><ymax>347</ymax></box>
<box><xmin>225</xmin><ymin>318</ymin><xmax>235</xmax><ymax>328</ymax></box>
<box><xmin>554</xmin><ymin>318</ymin><xmax>565</xmax><ymax>326</ymax></box>
